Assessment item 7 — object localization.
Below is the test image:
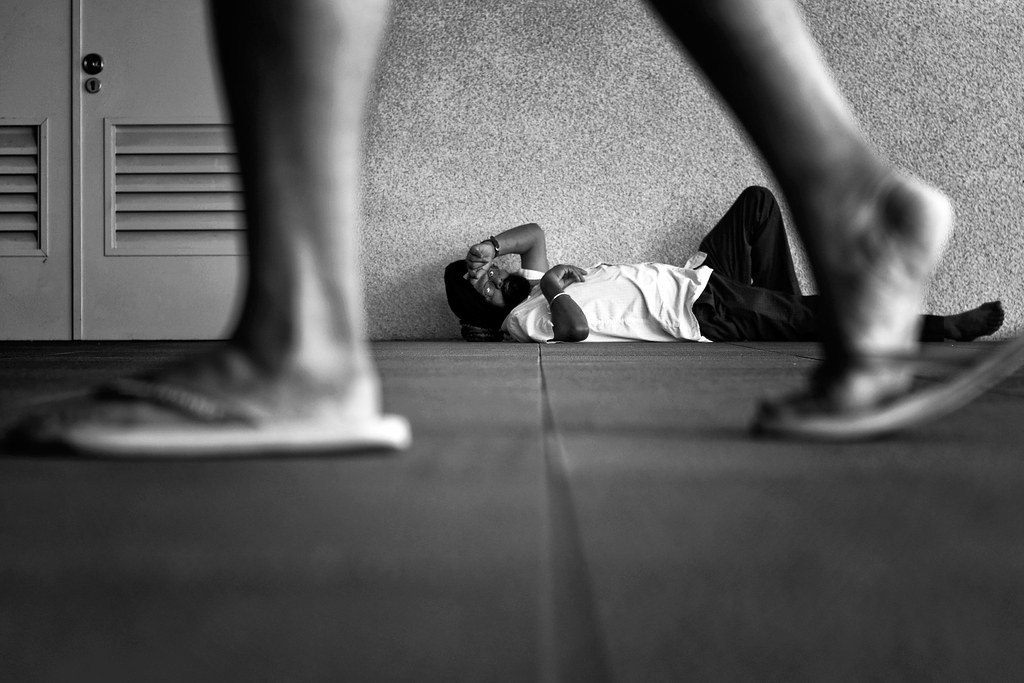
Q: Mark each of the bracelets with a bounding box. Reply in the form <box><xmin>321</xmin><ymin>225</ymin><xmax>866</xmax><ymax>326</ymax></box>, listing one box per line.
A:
<box><xmin>480</xmin><ymin>235</ymin><xmax>501</xmax><ymax>260</ymax></box>
<box><xmin>550</xmin><ymin>293</ymin><xmax>571</xmax><ymax>309</ymax></box>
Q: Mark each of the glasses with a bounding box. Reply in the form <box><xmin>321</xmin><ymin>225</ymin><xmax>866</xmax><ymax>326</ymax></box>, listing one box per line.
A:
<box><xmin>483</xmin><ymin>267</ymin><xmax>501</xmax><ymax>300</ymax></box>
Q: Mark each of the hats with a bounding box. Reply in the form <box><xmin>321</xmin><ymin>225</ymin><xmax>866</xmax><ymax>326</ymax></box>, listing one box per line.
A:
<box><xmin>443</xmin><ymin>259</ymin><xmax>511</xmax><ymax>330</ymax></box>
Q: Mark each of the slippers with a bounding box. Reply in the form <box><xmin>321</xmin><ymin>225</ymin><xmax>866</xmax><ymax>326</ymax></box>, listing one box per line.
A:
<box><xmin>751</xmin><ymin>330</ymin><xmax>1024</xmax><ymax>435</ymax></box>
<box><xmin>18</xmin><ymin>360</ymin><xmax>410</xmax><ymax>457</ymax></box>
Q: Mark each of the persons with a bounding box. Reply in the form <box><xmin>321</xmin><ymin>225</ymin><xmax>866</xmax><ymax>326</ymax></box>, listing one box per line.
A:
<box><xmin>23</xmin><ymin>0</ymin><xmax>1024</xmax><ymax>452</ymax></box>
<box><xmin>444</xmin><ymin>187</ymin><xmax>1004</xmax><ymax>343</ymax></box>
